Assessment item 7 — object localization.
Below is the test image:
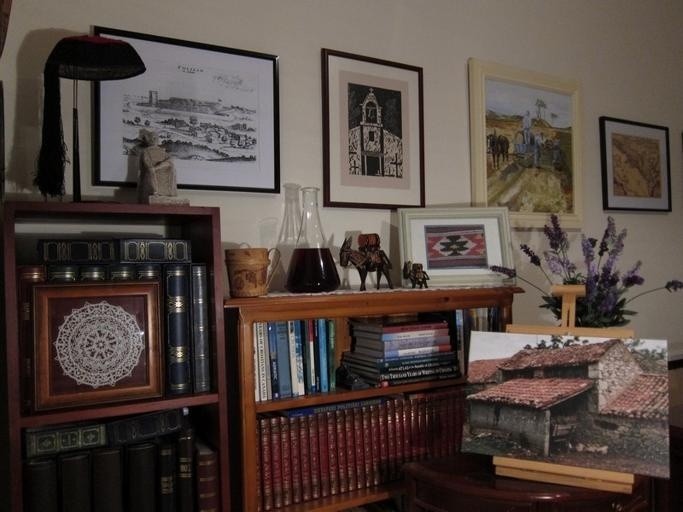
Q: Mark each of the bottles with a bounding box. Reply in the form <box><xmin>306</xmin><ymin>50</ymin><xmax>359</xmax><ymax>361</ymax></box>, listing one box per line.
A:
<box><xmin>286</xmin><ymin>187</ymin><xmax>340</xmax><ymax>294</ymax></box>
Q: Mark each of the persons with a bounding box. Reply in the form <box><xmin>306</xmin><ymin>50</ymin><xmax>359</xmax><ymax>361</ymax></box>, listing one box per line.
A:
<box><xmin>486</xmin><ymin>105</ymin><xmax>571</xmax><ymax>178</ymax></box>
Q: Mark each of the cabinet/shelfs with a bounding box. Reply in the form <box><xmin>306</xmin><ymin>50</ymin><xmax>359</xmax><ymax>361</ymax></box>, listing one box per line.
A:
<box><xmin>89</xmin><ymin>23</ymin><xmax>285</xmax><ymax>195</ymax></box>
<box><xmin>224</xmin><ymin>284</ymin><xmax>526</xmax><ymax>512</ymax></box>
<box><xmin>1</xmin><ymin>200</ymin><xmax>234</xmax><ymax>512</ymax></box>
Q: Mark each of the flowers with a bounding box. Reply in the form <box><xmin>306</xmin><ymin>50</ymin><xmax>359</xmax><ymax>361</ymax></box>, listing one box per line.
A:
<box><xmin>486</xmin><ymin>215</ymin><xmax>683</xmax><ymax>328</ymax></box>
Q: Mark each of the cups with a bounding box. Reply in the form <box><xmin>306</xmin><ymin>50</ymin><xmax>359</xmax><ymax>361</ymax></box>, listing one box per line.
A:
<box><xmin>224</xmin><ymin>247</ymin><xmax>281</xmax><ymax>298</ymax></box>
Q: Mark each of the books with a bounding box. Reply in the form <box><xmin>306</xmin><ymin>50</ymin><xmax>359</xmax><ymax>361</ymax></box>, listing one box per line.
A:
<box><xmin>253</xmin><ymin>306</ymin><xmax>506</xmax><ymax>510</ymax></box>
<box><xmin>16</xmin><ymin>216</ymin><xmax>222</xmax><ymax>510</ymax></box>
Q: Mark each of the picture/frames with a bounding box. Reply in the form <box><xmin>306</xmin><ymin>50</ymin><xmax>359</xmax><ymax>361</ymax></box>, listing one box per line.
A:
<box><xmin>393</xmin><ymin>204</ymin><xmax>518</xmax><ymax>288</ymax></box>
<box><xmin>467</xmin><ymin>55</ymin><xmax>586</xmax><ymax>231</ymax></box>
<box><xmin>598</xmin><ymin>114</ymin><xmax>672</xmax><ymax>212</ymax></box>
<box><xmin>318</xmin><ymin>47</ymin><xmax>426</xmax><ymax>211</ymax></box>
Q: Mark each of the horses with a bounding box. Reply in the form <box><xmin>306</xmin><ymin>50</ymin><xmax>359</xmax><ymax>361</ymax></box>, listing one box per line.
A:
<box><xmin>487</xmin><ymin>134</ymin><xmax>509</xmax><ymax>170</ymax></box>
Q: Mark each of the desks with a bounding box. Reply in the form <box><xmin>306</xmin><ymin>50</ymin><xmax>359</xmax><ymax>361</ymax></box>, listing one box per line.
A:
<box><xmin>394</xmin><ymin>455</ymin><xmax>658</xmax><ymax>511</ymax></box>
